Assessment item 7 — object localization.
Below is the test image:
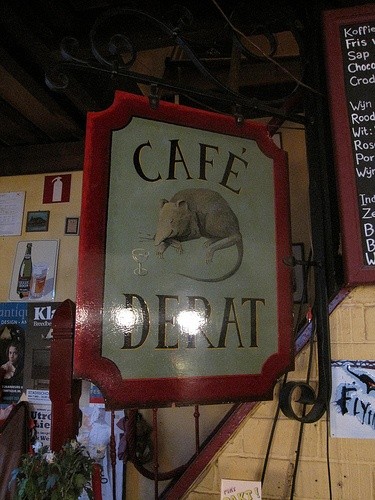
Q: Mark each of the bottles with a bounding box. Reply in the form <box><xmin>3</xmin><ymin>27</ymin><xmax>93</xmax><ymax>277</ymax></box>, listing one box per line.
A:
<box><xmin>17</xmin><ymin>243</ymin><xmax>32</xmax><ymax>295</ymax></box>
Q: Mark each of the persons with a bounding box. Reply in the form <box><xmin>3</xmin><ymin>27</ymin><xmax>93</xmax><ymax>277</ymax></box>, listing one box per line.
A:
<box><xmin>0</xmin><ymin>343</ymin><xmax>23</xmax><ymax>384</ymax></box>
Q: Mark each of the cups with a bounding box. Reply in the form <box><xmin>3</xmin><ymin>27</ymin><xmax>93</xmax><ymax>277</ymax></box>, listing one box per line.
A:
<box><xmin>30</xmin><ymin>263</ymin><xmax>48</xmax><ymax>297</ymax></box>
